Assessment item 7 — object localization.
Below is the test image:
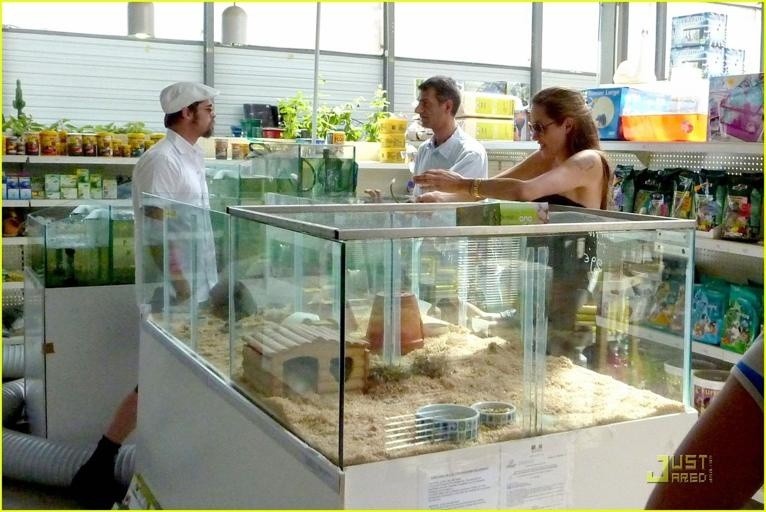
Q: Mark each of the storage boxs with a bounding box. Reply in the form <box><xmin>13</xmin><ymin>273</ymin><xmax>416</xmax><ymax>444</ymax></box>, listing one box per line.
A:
<box><xmin>454</xmin><ymin>92</ymin><xmax>514</xmax><ymax>119</ymax></box>
<box><xmin>464</xmin><ymin>117</ymin><xmax>514</xmax><ymax>142</ymax></box>
<box><xmin>619</xmin><ymin>113</ymin><xmax>707</xmax><ymax>143</ymax></box>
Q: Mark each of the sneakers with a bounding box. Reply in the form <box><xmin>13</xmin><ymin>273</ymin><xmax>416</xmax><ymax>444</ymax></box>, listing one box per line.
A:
<box><xmin>66</xmin><ymin>463</ymin><xmax>128</xmax><ymax>510</ymax></box>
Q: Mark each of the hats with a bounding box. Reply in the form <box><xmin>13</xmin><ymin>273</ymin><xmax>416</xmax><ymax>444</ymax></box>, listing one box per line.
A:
<box><xmin>157</xmin><ymin>79</ymin><xmax>222</xmax><ymax>116</ymax></box>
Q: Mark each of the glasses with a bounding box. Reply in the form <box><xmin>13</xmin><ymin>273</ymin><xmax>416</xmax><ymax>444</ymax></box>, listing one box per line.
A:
<box><xmin>525</xmin><ymin>119</ymin><xmax>565</xmax><ymax>137</ymax></box>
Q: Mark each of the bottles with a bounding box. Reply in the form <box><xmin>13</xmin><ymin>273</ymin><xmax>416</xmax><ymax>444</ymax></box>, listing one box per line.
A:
<box><xmin>609</xmin><ymin>337</ymin><xmax>646</xmax><ymax>388</ymax></box>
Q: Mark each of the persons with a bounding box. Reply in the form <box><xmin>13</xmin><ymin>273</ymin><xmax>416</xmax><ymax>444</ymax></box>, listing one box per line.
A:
<box><xmin>641</xmin><ymin>329</ymin><xmax>762</xmax><ymax>510</ymax></box>
<box><xmin>449</xmin><ymin>263</ymin><xmax>521</xmax><ymax>340</ymax></box>
<box><xmin>67</xmin><ymin>276</ymin><xmax>258</xmax><ymax>509</ymax></box>
<box><xmin>362</xmin><ymin>72</ymin><xmax>490</xmax><ymax>302</ymax></box>
<box><xmin>411</xmin><ymin>85</ymin><xmax>611</xmax><ymax>359</ymax></box>
<box><xmin>132</xmin><ymin>81</ymin><xmax>219</xmax><ymax>315</ymax></box>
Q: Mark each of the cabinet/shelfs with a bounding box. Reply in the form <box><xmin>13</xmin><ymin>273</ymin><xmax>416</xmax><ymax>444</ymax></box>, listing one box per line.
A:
<box><xmin>133</xmin><ymin>190</ymin><xmax>698</xmax><ymax>510</ymax></box>
<box><xmin>476</xmin><ymin>140</ymin><xmax>764</xmax><ymax>509</ymax></box>
<box><xmin>2</xmin><ymin>133</ymin><xmax>411</xmax><ymax>289</ymax></box>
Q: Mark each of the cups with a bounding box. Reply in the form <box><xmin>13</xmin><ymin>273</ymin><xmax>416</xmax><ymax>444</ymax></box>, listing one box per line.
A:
<box><xmin>228</xmin><ymin>119</ymin><xmax>281</xmax><ymax>138</ymax></box>
<box><xmin>325</xmin><ymin>132</ymin><xmax>344</xmax><ymax>144</ymax></box>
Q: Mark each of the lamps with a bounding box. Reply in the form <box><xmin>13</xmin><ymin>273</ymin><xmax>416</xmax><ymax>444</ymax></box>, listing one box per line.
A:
<box><xmin>128</xmin><ymin>2</ymin><xmax>154</xmax><ymax>40</ymax></box>
<box><xmin>221</xmin><ymin>2</ymin><xmax>247</xmax><ymax>46</ymax></box>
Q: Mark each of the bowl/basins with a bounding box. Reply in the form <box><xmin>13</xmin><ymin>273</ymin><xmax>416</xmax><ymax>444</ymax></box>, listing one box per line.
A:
<box><xmin>415</xmin><ymin>404</ymin><xmax>481</xmax><ymax>443</ymax></box>
<box><xmin>471</xmin><ymin>402</ymin><xmax>515</xmax><ymax>427</ymax></box>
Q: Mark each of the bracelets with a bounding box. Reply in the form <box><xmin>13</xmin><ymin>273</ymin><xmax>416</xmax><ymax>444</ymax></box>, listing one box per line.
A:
<box><xmin>472</xmin><ymin>177</ymin><xmax>485</xmax><ymax>199</ymax></box>
